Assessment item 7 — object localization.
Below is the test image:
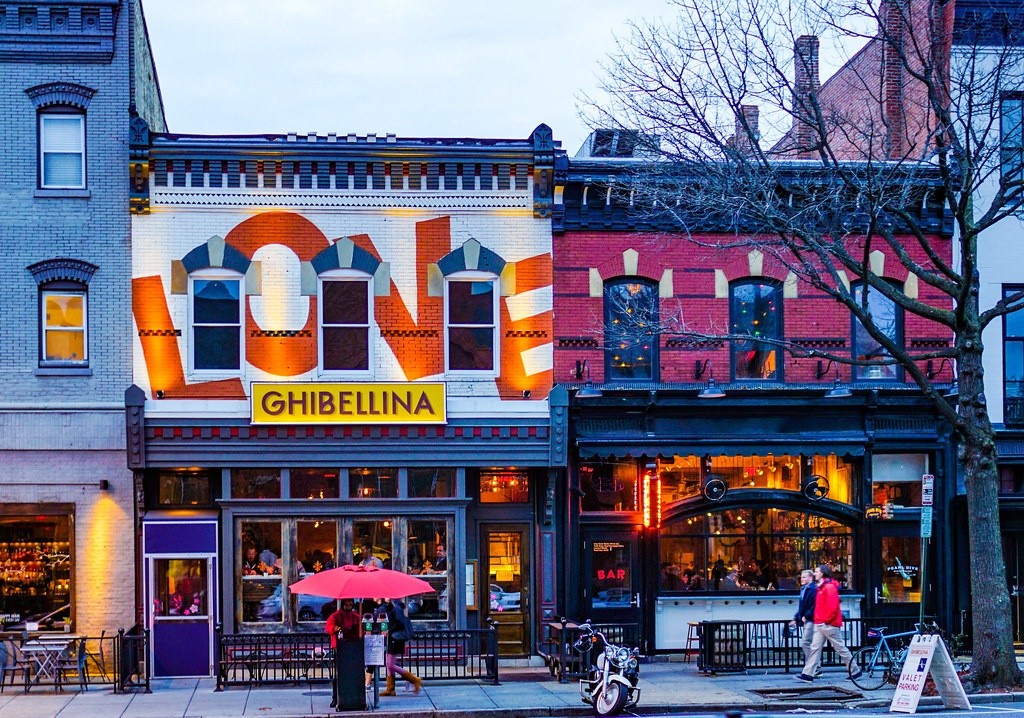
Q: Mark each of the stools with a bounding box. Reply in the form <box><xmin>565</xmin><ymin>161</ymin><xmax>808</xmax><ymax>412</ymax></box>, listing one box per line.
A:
<box><xmin>683</xmin><ymin>618</ymin><xmax>805</xmax><ymax>668</ymax></box>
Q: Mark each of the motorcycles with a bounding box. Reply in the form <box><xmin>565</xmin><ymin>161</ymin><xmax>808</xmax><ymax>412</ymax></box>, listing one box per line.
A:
<box><xmin>574</xmin><ymin>618</ymin><xmax>641</xmax><ymax>718</ymax></box>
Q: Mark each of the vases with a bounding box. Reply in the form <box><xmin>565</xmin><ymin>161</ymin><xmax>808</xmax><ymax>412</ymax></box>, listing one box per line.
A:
<box><xmin>263</xmin><ymin>572</ymin><xmax>269</xmax><ymax>576</ymax></box>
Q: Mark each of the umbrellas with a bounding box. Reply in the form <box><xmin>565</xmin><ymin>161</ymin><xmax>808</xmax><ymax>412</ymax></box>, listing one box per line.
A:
<box><xmin>288</xmin><ymin>565</ymin><xmax>436</xmax><ymax>638</ymax></box>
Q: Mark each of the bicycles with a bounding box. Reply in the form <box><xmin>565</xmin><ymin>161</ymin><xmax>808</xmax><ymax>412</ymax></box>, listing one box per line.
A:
<box><xmin>847</xmin><ymin>621</ymin><xmax>940</xmax><ymax>691</ymax></box>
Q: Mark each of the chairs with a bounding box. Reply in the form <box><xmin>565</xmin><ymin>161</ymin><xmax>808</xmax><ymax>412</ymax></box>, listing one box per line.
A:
<box><xmin>0</xmin><ymin>629</ymin><xmax>105</xmax><ymax>696</ymax></box>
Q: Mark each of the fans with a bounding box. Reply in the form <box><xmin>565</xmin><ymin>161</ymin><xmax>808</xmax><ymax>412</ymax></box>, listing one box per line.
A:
<box><xmin>699</xmin><ymin>474</ymin><xmax>729</xmax><ymax>502</ymax></box>
<box><xmin>804</xmin><ymin>473</ymin><xmax>830</xmax><ymax>500</ymax></box>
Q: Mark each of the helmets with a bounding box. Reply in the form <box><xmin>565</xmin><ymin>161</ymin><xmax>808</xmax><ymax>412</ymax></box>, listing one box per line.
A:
<box><xmin>574</xmin><ymin>637</ymin><xmax>593</xmax><ymax>652</ymax></box>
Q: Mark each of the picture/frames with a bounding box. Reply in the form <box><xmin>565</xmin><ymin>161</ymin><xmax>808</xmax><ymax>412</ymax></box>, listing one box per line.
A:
<box><xmin>847</xmin><ymin>554</ymin><xmax>853</xmax><ymax>566</ymax></box>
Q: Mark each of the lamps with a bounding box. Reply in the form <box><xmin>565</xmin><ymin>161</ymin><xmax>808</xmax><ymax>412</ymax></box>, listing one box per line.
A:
<box><xmin>755</xmin><ymin>458</ymin><xmax>764</xmax><ymax>475</ymax></box>
<box><xmin>100</xmin><ymin>479</ymin><xmax>110</xmax><ymax>491</ymax></box>
<box><xmin>784</xmin><ymin>456</ymin><xmax>793</xmax><ymax>470</ymax></box>
<box><xmin>816</xmin><ymin>360</ymin><xmax>852</xmax><ymax>398</ymax></box>
<box><xmin>768</xmin><ymin>454</ymin><xmax>777</xmax><ymax>472</ymax></box>
<box><xmin>744</xmin><ymin>458</ymin><xmax>748</xmax><ymax>478</ymax></box>
<box><xmin>569</xmin><ymin>359</ymin><xmax>601</xmax><ymax>397</ymax></box>
<box><xmin>796</xmin><ymin>454</ymin><xmax>802</xmax><ymax>465</ymax></box>
<box><xmin>693</xmin><ymin>357</ymin><xmax>727</xmax><ymax>397</ymax></box>
<box><xmin>927</xmin><ymin>355</ymin><xmax>960</xmax><ymax>398</ymax></box>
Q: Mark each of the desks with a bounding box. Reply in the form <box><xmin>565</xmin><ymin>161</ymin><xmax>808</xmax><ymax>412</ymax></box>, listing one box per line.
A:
<box><xmin>409</xmin><ymin>572</ymin><xmax>448</xmax><ymax>577</ymax></box>
<box><xmin>26</xmin><ymin>640</ymin><xmax>71</xmax><ymax>684</ymax></box>
<box><xmin>20</xmin><ymin>645</ymin><xmax>67</xmax><ymax>694</ymax></box>
<box><xmin>39</xmin><ymin>632</ymin><xmax>85</xmax><ymax>682</ymax></box>
<box><xmin>243</xmin><ymin>572</ymin><xmax>316</xmax><ymax>583</ymax></box>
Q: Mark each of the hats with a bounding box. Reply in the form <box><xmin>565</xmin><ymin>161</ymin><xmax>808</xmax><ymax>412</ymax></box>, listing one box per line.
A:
<box><xmin>819</xmin><ymin>565</ymin><xmax>832</xmax><ymax>577</ymax></box>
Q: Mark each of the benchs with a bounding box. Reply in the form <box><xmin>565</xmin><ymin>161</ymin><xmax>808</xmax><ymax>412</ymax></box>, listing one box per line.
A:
<box><xmin>220</xmin><ymin>645</ymin><xmax>463</xmax><ymax>688</ymax></box>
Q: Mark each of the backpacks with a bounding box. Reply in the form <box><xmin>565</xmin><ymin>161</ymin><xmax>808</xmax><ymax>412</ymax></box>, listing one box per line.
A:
<box><xmin>392</xmin><ymin>599</ymin><xmax>414</xmax><ymax>639</ymax></box>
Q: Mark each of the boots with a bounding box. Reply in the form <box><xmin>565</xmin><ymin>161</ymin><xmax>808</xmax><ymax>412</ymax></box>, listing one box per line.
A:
<box><xmin>379</xmin><ymin>676</ymin><xmax>395</xmax><ymax>696</ymax></box>
<box><xmin>402</xmin><ymin>670</ymin><xmax>421</xmax><ymax>692</ymax></box>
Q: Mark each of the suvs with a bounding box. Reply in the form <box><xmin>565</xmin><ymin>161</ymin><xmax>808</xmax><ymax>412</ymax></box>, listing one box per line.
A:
<box><xmin>437</xmin><ymin>582</ymin><xmax>515</xmax><ymax>613</ymax></box>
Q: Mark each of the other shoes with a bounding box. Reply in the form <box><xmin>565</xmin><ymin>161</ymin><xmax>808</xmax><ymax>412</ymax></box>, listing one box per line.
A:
<box><xmin>845</xmin><ymin>671</ymin><xmax>863</xmax><ymax>681</ymax></box>
<box><xmin>365</xmin><ymin>685</ymin><xmax>371</xmax><ymax>692</ymax></box>
<box><xmin>330</xmin><ymin>699</ymin><xmax>337</xmax><ymax>707</ymax></box>
<box><xmin>793</xmin><ymin>674</ymin><xmax>813</xmax><ymax>683</ymax></box>
<box><xmin>815</xmin><ymin>670</ymin><xmax>823</xmax><ymax>676</ymax></box>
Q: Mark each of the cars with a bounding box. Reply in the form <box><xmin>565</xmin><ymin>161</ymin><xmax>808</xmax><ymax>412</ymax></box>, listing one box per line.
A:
<box><xmin>5</xmin><ymin>603</ymin><xmax>70</xmax><ymax>631</ymax></box>
<box><xmin>257</xmin><ymin>588</ymin><xmax>418</xmax><ymax>621</ymax></box>
<box><xmin>592</xmin><ymin>588</ymin><xmax>632</xmax><ymax>607</ymax></box>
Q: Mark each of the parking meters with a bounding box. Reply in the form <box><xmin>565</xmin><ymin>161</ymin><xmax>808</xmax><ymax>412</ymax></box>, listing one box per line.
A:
<box><xmin>362</xmin><ymin>613</ymin><xmax>389</xmax><ymax>708</ymax></box>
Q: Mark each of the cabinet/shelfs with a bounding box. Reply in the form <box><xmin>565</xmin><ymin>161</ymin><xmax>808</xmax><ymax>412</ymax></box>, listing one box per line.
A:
<box><xmin>771</xmin><ymin>525</ymin><xmax>847</xmax><ymax>573</ymax></box>
<box><xmin>547</xmin><ymin>621</ymin><xmax>582</xmax><ymax>682</ymax></box>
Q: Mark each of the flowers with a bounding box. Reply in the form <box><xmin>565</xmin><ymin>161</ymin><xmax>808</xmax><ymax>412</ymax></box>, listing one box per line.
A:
<box><xmin>259</xmin><ymin>562</ymin><xmax>274</xmax><ymax>572</ymax></box>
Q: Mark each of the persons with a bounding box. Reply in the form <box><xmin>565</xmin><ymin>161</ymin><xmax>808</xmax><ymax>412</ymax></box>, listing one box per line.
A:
<box><xmin>242</xmin><ymin>545</ymin><xmax>263</xmax><ymax>576</ymax></box>
<box><xmin>325</xmin><ymin>598</ymin><xmax>361</xmax><ymax>708</ymax></box>
<box><xmin>365</xmin><ymin>598</ymin><xmax>422</xmax><ymax>696</ymax></box>
<box><xmin>433</xmin><ymin>544</ymin><xmax>447</xmax><ymax>571</ymax></box>
<box><xmin>661</xmin><ymin>558</ymin><xmax>779</xmax><ymax>591</ymax></box>
<box><xmin>789</xmin><ymin>565</ymin><xmax>862</xmax><ymax>682</ymax></box>
<box><xmin>301</xmin><ymin>543</ymin><xmax>383</xmax><ymax>570</ymax></box>
